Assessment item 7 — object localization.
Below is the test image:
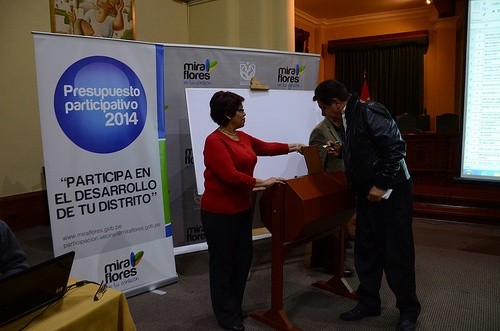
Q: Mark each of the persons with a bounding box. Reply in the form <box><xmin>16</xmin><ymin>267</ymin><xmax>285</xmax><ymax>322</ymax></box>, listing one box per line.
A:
<box><xmin>312</xmin><ymin>80</ymin><xmax>421</xmax><ymax>331</ymax></box>
<box><xmin>0</xmin><ymin>221</ymin><xmax>28</xmax><ymax>280</ymax></box>
<box><xmin>309</xmin><ymin>117</ymin><xmax>357</xmax><ymax>277</ymax></box>
<box><xmin>200</xmin><ymin>91</ymin><xmax>306</xmax><ymax>331</ymax></box>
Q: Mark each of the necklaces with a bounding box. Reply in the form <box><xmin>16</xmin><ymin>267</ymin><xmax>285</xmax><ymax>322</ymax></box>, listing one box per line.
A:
<box><xmin>220</xmin><ymin>128</ymin><xmax>237</xmax><ymax>136</ymax></box>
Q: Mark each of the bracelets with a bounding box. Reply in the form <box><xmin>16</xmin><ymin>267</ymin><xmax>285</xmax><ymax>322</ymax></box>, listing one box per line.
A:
<box><xmin>296</xmin><ymin>143</ymin><xmax>299</xmax><ymax>153</ymax></box>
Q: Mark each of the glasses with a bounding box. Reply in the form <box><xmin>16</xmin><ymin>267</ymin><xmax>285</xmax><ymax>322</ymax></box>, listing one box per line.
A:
<box><xmin>236</xmin><ymin>108</ymin><xmax>245</xmax><ymax>115</ymax></box>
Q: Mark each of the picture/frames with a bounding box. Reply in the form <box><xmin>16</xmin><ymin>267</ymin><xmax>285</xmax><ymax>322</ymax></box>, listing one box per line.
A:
<box><xmin>49</xmin><ymin>0</ymin><xmax>137</xmax><ymax>40</ymax></box>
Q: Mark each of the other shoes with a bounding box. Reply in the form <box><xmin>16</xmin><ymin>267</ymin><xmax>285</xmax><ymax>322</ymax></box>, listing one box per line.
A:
<box><xmin>223</xmin><ymin>305</ymin><xmax>247</xmax><ymax>330</ymax></box>
<box><xmin>396</xmin><ymin>316</ymin><xmax>417</xmax><ymax>331</ymax></box>
<box><xmin>340</xmin><ymin>305</ymin><xmax>382</xmax><ymax>321</ymax></box>
<box><xmin>314</xmin><ymin>262</ymin><xmax>355</xmax><ymax>276</ymax></box>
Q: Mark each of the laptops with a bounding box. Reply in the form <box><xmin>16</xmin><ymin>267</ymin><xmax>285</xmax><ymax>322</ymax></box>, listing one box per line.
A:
<box><xmin>0</xmin><ymin>250</ymin><xmax>75</xmax><ymax>327</ymax></box>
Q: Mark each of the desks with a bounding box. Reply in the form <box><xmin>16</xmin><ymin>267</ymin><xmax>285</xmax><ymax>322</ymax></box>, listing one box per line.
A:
<box><xmin>0</xmin><ymin>276</ymin><xmax>137</xmax><ymax>331</ymax></box>
